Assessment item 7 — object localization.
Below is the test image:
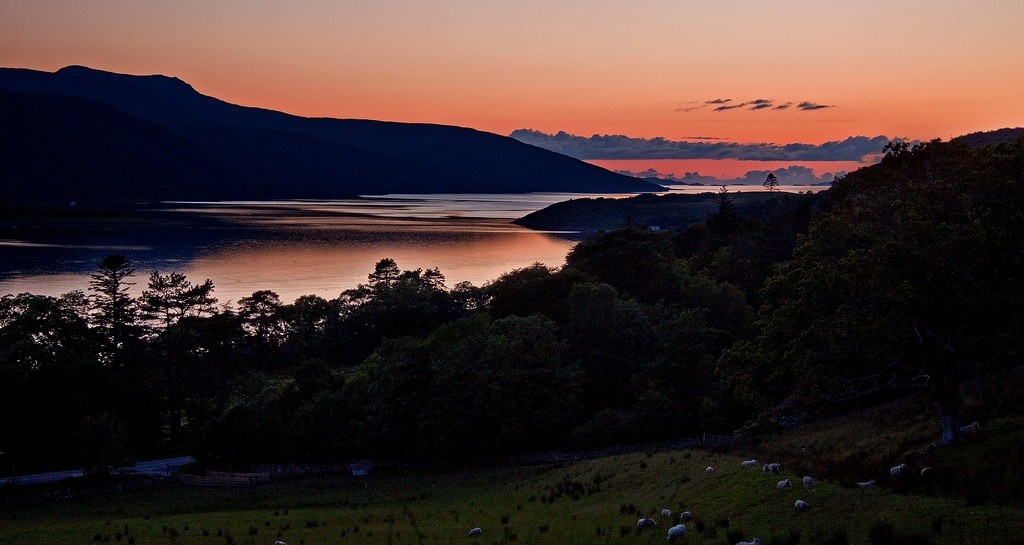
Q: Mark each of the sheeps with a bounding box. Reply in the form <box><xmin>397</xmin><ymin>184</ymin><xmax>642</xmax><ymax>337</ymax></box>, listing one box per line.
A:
<box><xmin>681</xmin><ymin>510</ymin><xmax>691</xmax><ymax>522</ymax></box>
<box><xmin>795</xmin><ymin>499</ymin><xmax>809</xmax><ymax>513</ymax></box>
<box><xmin>735</xmin><ymin>538</ymin><xmax>761</xmax><ymax>545</ymax></box>
<box><xmin>661</xmin><ymin>508</ymin><xmax>672</xmax><ymax>518</ymax></box>
<box><xmin>960</xmin><ymin>421</ymin><xmax>979</xmax><ymax>434</ymax></box>
<box><xmin>890</xmin><ymin>463</ymin><xmax>934</xmax><ymax>478</ymax></box>
<box><xmin>666</xmin><ymin>524</ymin><xmax>686</xmax><ymax>545</ymax></box>
<box><xmin>636</xmin><ymin>518</ymin><xmax>658</xmax><ymax>528</ymax></box>
<box><xmin>803</xmin><ymin>475</ymin><xmax>815</xmax><ymax>488</ymax></box>
<box><xmin>857</xmin><ymin>480</ymin><xmax>876</xmax><ymax>495</ymax></box>
<box><xmin>763</xmin><ymin>463</ymin><xmax>782</xmax><ymax>477</ymax></box>
<box><xmin>777</xmin><ymin>478</ymin><xmax>792</xmax><ymax>492</ymax></box>
<box><xmin>742</xmin><ymin>459</ymin><xmax>757</xmax><ymax>468</ymax></box>
<box><xmin>706</xmin><ymin>466</ymin><xmax>714</xmax><ymax>473</ymax></box>
